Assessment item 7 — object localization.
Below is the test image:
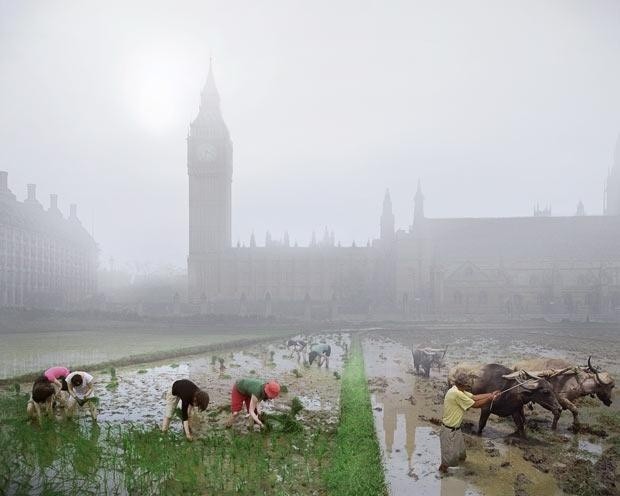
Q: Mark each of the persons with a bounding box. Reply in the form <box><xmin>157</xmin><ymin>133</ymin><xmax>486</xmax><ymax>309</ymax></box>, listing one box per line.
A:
<box><xmin>309</xmin><ymin>344</ymin><xmax>331</xmax><ymax>368</ymax></box>
<box><xmin>227</xmin><ymin>378</ymin><xmax>280</xmax><ymax>431</ymax></box>
<box><xmin>162</xmin><ymin>379</ymin><xmax>209</xmax><ymax>441</ymax></box>
<box><xmin>27</xmin><ymin>367</ymin><xmax>98</xmax><ymax>421</ymax></box>
<box><xmin>288</xmin><ymin>339</ymin><xmax>307</xmax><ymax>362</ymax></box>
<box><xmin>438</xmin><ymin>373</ymin><xmax>501</xmax><ymax>471</ymax></box>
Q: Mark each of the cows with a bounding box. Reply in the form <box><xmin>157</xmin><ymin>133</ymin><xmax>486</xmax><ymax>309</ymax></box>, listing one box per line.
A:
<box><xmin>448</xmin><ymin>362</ymin><xmax>564</xmax><ymax>439</ymax></box>
<box><xmin>411</xmin><ymin>342</ymin><xmax>447</xmax><ymax>376</ymax></box>
<box><xmin>514</xmin><ymin>354</ymin><xmax>616</xmax><ymax>435</ymax></box>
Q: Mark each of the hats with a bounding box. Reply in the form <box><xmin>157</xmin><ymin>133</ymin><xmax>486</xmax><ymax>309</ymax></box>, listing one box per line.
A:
<box><xmin>448</xmin><ymin>367</ymin><xmax>470</xmax><ymax>387</ymax></box>
<box><xmin>264</xmin><ymin>382</ymin><xmax>281</xmax><ymax>399</ymax></box>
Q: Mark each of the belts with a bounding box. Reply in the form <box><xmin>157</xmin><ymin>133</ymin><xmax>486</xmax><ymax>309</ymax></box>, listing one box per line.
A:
<box><xmin>443</xmin><ymin>424</ymin><xmax>461</xmax><ymax>432</ymax></box>
